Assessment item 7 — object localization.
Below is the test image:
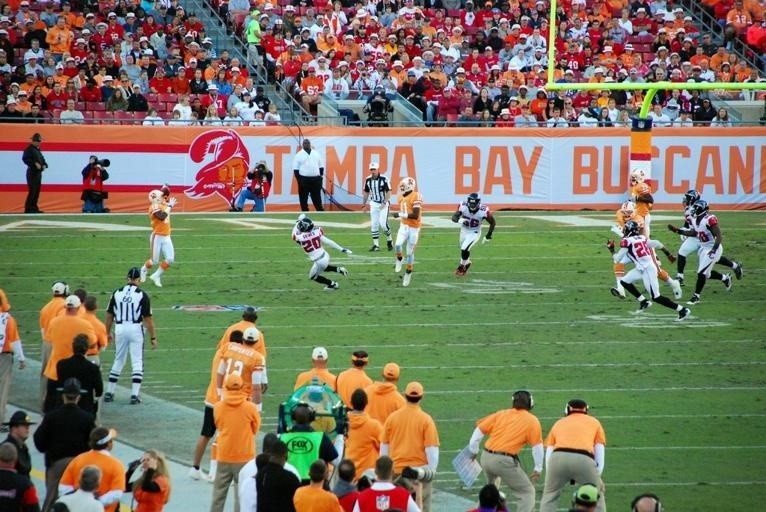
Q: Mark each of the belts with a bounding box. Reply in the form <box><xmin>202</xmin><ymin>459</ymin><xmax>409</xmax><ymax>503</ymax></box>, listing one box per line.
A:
<box><xmin>553</xmin><ymin>448</ymin><xmax>594</xmax><ymax>458</ymax></box>
<box><xmin>116</xmin><ymin>321</ymin><xmax>139</xmax><ymax>323</ymax></box>
<box><xmin>485</xmin><ymin>448</ymin><xmax>514</xmax><ymax>457</ymax></box>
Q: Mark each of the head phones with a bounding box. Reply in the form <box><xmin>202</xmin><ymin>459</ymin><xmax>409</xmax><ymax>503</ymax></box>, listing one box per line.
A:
<box><xmin>290</xmin><ymin>403</ymin><xmax>316</xmax><ymax>420</ymax></box>
<box><xmin>512</xmin><ymin>390</ymin><xmax>534</xmax><ymax>408</ymax></box>
<box><xmin>564</xmin><ymin>399</ymin><xmax>589</xmax><ymax>416</ymax></box>
<box><xmin>572</xmin><ymin>483</ymin><xmax>601</xmax><ymax>508</ymax></box>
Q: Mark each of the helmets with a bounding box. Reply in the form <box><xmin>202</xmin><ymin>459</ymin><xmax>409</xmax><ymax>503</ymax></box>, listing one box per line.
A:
<box><xmin>297</xmin><ymin>219</ymin><xmax>313</xmax><ymax>232</ymax></box>
<box><xmin>149</xmin><ymin>190</ymin><xmax>163</xmax><ymax>203</ymax></box>
<box><xmin>621</xmin><ymin>202</ymin><xmax>640</xmax><ymax>236</ymax></box>
<box><xmin>682</xmin><ymin>190</ymin><xmax>708</xmax><ymax>216</ymax></box>
<box><xmin>631</xmin><ymin>169</ymin><xmax>651</xmax><ymax>196</ymax></box>
<box><xmin>400</xmin><ymin>177</ymin><xmax>415</xmax><ymax>195</ymax></box>
<box><xmin>467</xmin><ymin>193</ymin><xmax>480</xmax><ymax>211</ymax></box>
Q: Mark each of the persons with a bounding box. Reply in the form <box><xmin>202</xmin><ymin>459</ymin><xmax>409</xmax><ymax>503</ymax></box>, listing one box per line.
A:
<box><xmin>80</xmin><ymin>155</ymin><xmax>110</xmax><ymax>212</ymax></box>
<box><xmin>0</xmin><ymin>0</ymin><xmax>765</xmax><ymax>128</ymax></box>
<box><xmin>291</xmin><ymin>214</ymin><xmax>352</xmax><ymax>292</ymax></box>
<box><xmin>607</xmin><ymin>167</ymin><xmax>743</xmax><ymax>322</ymax></box>
<box><xmin>0</xmin><ymin>269</ymin><xmax>171</xmax><ymax>511</ymax></box>
<box><xmin>21</xmin><ymin>132</ymin><xmax>47</xmax><ymax>213</ymax></box>
<box><xmin>390</xmin><ymin>177</ymin><xmax>421</xmax><ymax>287</ymax></box>
<box><xmin>451</xmin><ymin>192</ymin><xmax>496</xmax><ymax>275</ymax></box>
<box><xmin>137</xmin><ymin>183</ymin><xmax>178</xmax><ymax>287</ymax></box>
<box><xmin>190</xmin><ymin>307</ymin><xmax>440</xmax><ymax>511</ymax></box>
<box><xmin>465</xmin><ymin>391</ymin><xmax>608</xmax><ymax>511</ymax></box>
<box><xmin>293</xmin><ymin>138</ymin><xmax>324</xmax><ymax>211</ymax></box>
<box><xmin>235</xmin><ymin>161</ymin><xmax>272</xmax><ymax>212</ymax></box>
<box><xmin>361</xmin><ymin>162</ymin><xmax>394</xmax><ymax>251</ymax></box>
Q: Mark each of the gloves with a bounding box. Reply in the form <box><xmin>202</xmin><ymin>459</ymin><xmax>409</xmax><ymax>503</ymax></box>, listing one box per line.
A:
<box><xmin>607</xmin><ymin>240</ymin><xmax>614</xmax><ymax>249</ymax></box>
<box><xmin>168</xmin><ymin>197</ymin><xmax>177</xmax><ymax>207</ymax></box>
<box><xmin>482</xmin><ymin>235</ymin><xmax>490</xmax><ymax>244</ymax></box>
<box><xmin>458</xmin><ymin>218</ymin><xmax>466</xmax><ymax>224</ymax></box>
<box><xmin>342</xmin><ymin>249</ymin><xmax>352</xmax><ymax>254</ymax></box>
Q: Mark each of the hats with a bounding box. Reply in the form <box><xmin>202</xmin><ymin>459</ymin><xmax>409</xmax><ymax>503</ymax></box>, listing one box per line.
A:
<box><xmin>96</xmin><ymin>428</ymin><xmax>116</xmax><ymax>445</ymax></box>
<box><xmin>577</xmin><ymin>485</ymin><xmax>598</xmax><ymax>502</ymax></box>
<box><xmin>56</xmin><ymin>378</ymin><xmax>89</xmax><ymax>393</ymax></box>
<box><xmin>369</xmin><ymin>162</ymin><xmax>379</xmax><ymax>170</ymax></box>
<box><xmin>128</xmin><ymin>267</ymin><xmax>140</xmax><ymax>278</ymax></box>
<box><xmin>53</xmin><ymin>283</ymin><xmax>81</xmax><ymax>308</ymax></box>
<box><xmin>312</xmin><ymin>347</ymin><xmax>328</xmax><ymax>361</ymax></box>
<box><xmin>243</xmin><ymin>327</ymin><xmax>261</xmax><ymax>341</ymax></box>
<box><xmin>2</xmin><ymin>411</ymin><xmax>36</xmax><ymax>425</ymax></box>
<box><xmin>351</xmin><ymin>355</ymin><xmax>368</xmax><ymax>365</ymax></box>
<box><xmin>0</xmin><ymin>2</ymin><xmax>250</xmax><ymax>107</ymax></box>
<box><xmin>383</xmin><ymin>363</ymin><xmax>424</xmax><ymax>397</ymax></box>
<box><xmin>225</xmin><ymin>375</ymin><xmax>242</xmax><ymax>389</ymax></box>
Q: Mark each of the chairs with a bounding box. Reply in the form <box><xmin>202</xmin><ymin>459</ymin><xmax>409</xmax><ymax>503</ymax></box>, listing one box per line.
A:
<box><xmin>625</xmin><ymin>34</ymin><xmax>658</xmax><ymax>62</ymax></box>
<box><xmin>30</xmin><ymin>90</ymin><xmax>281</xmax><ymax>126</ymax></box>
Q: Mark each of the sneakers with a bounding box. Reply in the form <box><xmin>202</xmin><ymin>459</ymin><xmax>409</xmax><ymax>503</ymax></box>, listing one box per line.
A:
<box><xmin>723</xmin><ymin>262</ymin><xmax>743</xmax><ymax>290</ymax></box>
<box><xmin>140</xmin><ymin>271</ymin><xmax>162</xmax><ymax>287</ymax></box>
<box><xmin>369</xmin><ymin>240</ymin><xmax>393</xmax><ymax>251</ymax></box>
<box><xmin>104</xmin><ymin>392</ymin><xmax>114</xmax><ymax>401</ymax></box>
<box><xmin>396</xmin><ymin>258</ymin><xmax>412</xmax><ymax>287</ymax></box>
<box><xmin>131</xmin><ymin>395</ymin><xmax>141</xmax><ymax>404</ymax></box>
<box><xmin>611</xmin><ymin>287</ymin><xmax>626</xmax><ymax>299</ymax></box>
<box><xmin>456</xmin><ymin>261</ymin><xmax>471</xmax><ymax>275</ymax></box>
<box><xmin>324</xmin><ymin>281</ymin><xmax>338</xmax><ymax>289</ymax></box>
<box><xmin>189</xmin><ymin>467</ymin><xmax>215</xmax><ymax>482</ymax></box>
<box><xmin>337</xmin><ymin>267</ymin><xmax>348</xmax><ymax>277</ymax></box>
<box><xmin>673</xmin><ymin>277</ymin><xmax>700</xmax><ymax>321</ymax></box>
<box><xmin>638</xmin><ymin>300</ymin><xmax>652</xmax><ymax>313</ymax></box>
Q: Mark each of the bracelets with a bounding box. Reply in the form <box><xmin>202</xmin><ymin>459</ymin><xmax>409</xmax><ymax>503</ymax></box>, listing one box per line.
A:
<box><xmin>382</xmin><ymin>200</ymin><xmax>387</xmax><ymax>205</ymax></box>
<box><xmin>398</xmin><ymin>213</ymin><xmax>407</xmax><ymax>218</ymax></box>
<box><xmin>341</xmin><ymin>248</ymin><xmax>346</xmax><ymax>252</ymax></box>
